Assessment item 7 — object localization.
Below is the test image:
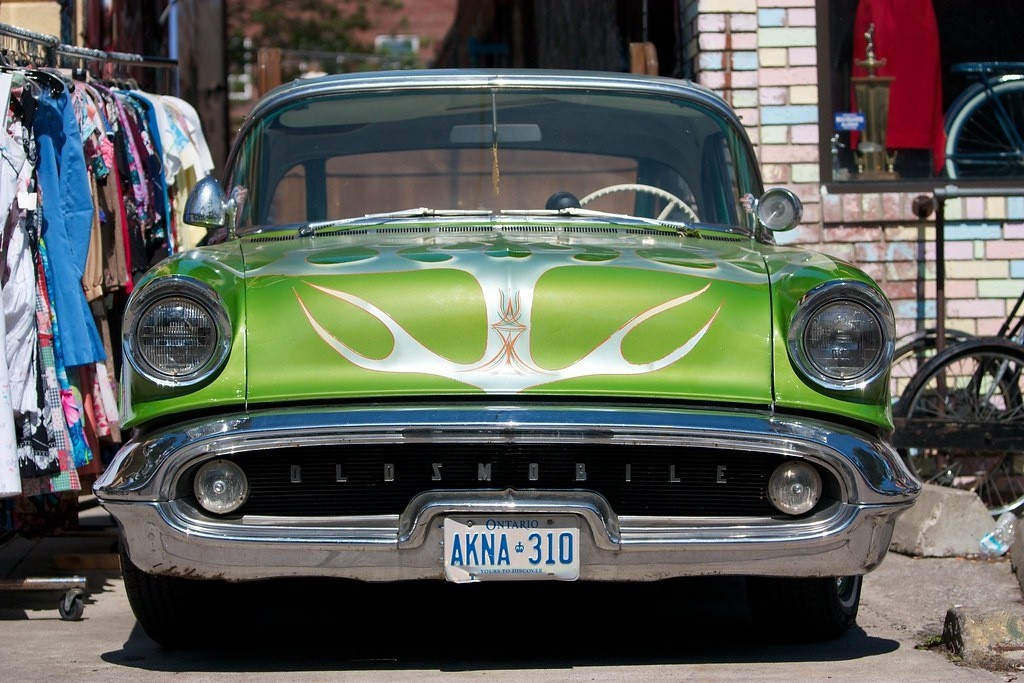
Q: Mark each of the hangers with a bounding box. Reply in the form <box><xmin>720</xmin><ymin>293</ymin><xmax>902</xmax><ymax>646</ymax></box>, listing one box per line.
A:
<box><xmin>0</xmin><ymin>23</ymin><xmax>143</xmax><ymax>98</ymax></box>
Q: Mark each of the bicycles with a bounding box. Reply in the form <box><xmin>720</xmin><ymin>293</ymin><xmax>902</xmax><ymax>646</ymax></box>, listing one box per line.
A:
<box><xmin>934</xmin><ymin>58</ymin><xmax>1024</xmax><ymax>194</ymax></box>
<box><xmin>891</xmin><ymin>290</ymin><xmax>1024</xmax><ymax>518</ymax></box>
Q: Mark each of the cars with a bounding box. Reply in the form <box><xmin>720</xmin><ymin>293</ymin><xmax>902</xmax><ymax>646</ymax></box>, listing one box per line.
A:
<box><xmin>91</xmin><ymin>66</ymin><xmax>922</xmax><ymax>648</ymax></box>
